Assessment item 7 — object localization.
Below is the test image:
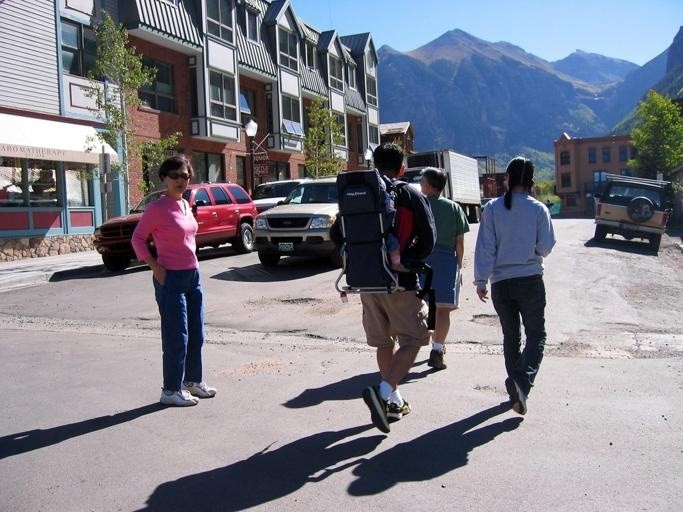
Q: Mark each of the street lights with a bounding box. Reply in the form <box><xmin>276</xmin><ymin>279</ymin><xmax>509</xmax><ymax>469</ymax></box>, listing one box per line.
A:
<box><xmin>244</xmin><ymin>119</ymin><xmax>258</xmax><ymax>197</ymax></box>
<box><xmin>363</xmin><ymin>149</ymin><xmax>372</xmax><ymax>170</ymax></box>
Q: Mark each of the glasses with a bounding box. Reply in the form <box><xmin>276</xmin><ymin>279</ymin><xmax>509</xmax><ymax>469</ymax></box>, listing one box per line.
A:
<box><xmin>167</xmin><ymin>172</ymin><xmax>191</xmax><ymax>180</ymax></box>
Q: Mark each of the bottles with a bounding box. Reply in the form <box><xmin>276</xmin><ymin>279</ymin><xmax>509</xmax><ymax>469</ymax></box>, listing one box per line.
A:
<box><xmin>385</xmin><ymin>235</ymin><xmax>401</xmax><ymax>266</ymax></box>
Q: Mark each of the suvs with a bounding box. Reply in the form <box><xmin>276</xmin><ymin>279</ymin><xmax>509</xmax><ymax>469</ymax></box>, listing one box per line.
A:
<box><xmin>92</xmin><ymin>180</ymin><xmax>257</xmax><ymax>272</ymax></box>
<box><xmin>252</xmin><ymin>176</ymin><xmax>341</xmax><ymax>267</ymax></box>
<box><xmin>593</xmin><ymin>173</ymin><xmax>675</xmax><ymax>251</ymax></box>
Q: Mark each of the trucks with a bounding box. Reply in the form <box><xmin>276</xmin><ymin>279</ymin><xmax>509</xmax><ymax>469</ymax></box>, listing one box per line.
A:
<box><xmin>401</xmin><ymin>149</ymin><xmax>481</xmax><ymax>224</ymax></box>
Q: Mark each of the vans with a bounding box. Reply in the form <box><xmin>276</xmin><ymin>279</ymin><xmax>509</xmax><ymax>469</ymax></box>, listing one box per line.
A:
<box><xmin>249</xmin><ymin>178</ymin><xmax>308</xmax><ymax>213</ymax></box>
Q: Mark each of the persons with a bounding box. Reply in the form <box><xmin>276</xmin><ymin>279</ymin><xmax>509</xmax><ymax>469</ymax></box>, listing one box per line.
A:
<box><xmin>329</xmin><ymin>141</ymin><xmax>438</xmax><ymax>436</ymax></box>
<box><xmin>473</xmin><ymin>157</ymin><xmax>557</xmax><ymax>417</ymax></box>
<box><xmin>413</xmin><ymin>168</ymin><xmax>470</xmax><ymax>370</ymax></box>
<box><xmin>131</xmin><ymin>153</ymin><xmax>217</xmax><ymax>407</ymax></box>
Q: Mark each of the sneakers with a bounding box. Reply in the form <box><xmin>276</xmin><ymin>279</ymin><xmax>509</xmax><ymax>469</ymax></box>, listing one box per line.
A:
<box><xmin>427</xmin><ymin>350</ymin><xmax>446</xmax><ymax>369</ymax></box>
<box><xmin>362</xmin><ymin>385</ymin><xmax>390</xmax><ymax>433</ymax></box>
<box><xmin>160</xmin><ymin>389</ymin><xmax>199</xmax><ymax>406</ymax></box>
<box><xmin>386</xmin><ymin>398</ymin><xmax>410</xmax><ymax>420</ymax></box>
<box><xmin>390</xmin><ymin>264</ymin><xmax>411</xmax><ymax>273</ymax></box>
<box><xmin>182</xmin><ymin>381</ymin><xmax>217</xmax><ymax>398</ymax></box>
<box><xmin>504</xmin><ymin>377</ymin><xmax>527</xmax><ymax>414</ymax></box>
